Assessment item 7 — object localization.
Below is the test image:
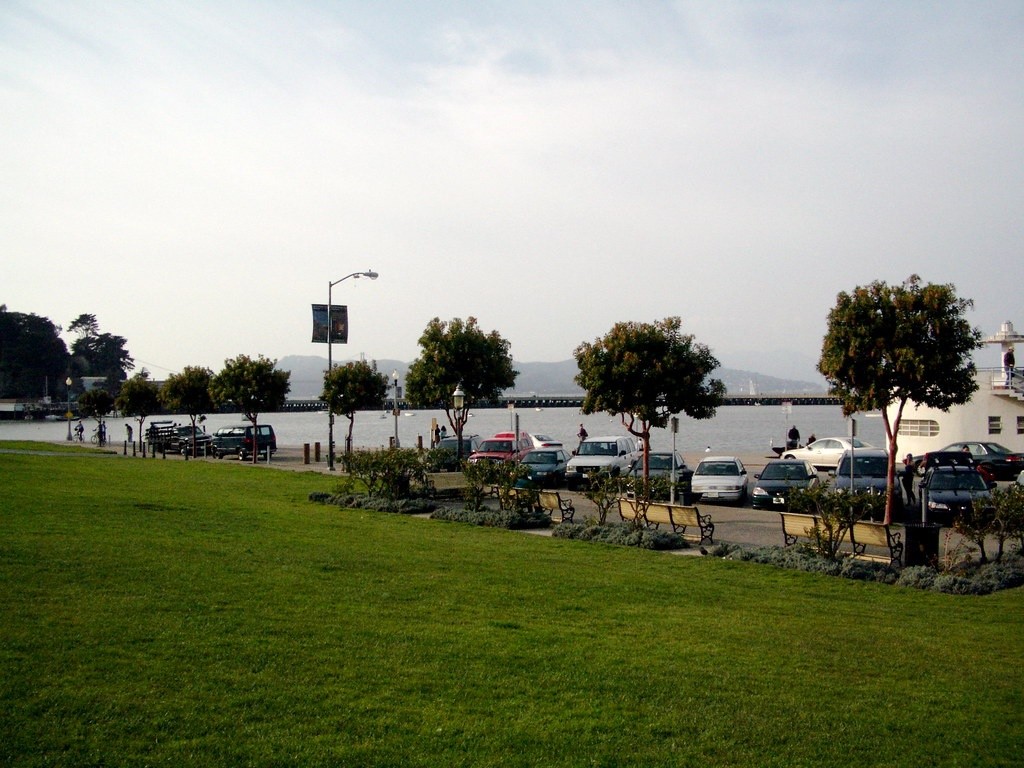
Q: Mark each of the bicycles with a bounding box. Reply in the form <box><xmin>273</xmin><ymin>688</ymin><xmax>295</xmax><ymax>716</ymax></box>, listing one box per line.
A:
<box><xmin>90</xmin><ymin>427</ymin><xmax>107</xmax><ymax>446</ymax></box>
<box><xmin>73</xmin><ymin>432</ymin><xmax>86</xmax><ymax>444</ymax></box>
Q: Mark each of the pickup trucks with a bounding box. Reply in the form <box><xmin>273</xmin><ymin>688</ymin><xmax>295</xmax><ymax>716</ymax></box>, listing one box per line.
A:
<box><xmin>166</xmin><ymin>425</ymin><xmax>212</xmax><ymax>457</ymax></box>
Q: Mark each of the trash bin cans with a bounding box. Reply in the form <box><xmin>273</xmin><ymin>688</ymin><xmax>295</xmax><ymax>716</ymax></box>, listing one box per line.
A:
<box><xmin>677</xmin><ymin>487</ymin><xmax>691</xmax><ymax>507</ymax></box>
<box><xmin>901</xmin><ymin>521</ymin><xmax>943</xmax><ymax>571</ymax></box>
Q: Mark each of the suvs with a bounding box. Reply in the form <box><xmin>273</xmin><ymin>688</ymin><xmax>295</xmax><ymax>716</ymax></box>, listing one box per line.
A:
<box><xmin>433</xmin><ymin>434</ymin><xmax>485</xmax><ymax>472</ymax></box>
<box><xmin>564</xmin><ymin>435</ymin><xmax>638</xmax><ymax>490</ymax></box>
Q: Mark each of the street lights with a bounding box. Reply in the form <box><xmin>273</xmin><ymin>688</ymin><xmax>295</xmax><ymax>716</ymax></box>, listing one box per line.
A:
<box><xmin>391</xmin><ymin>368</ymin><xmax>401</xmax><ymax>438</ymax></box>
<box><xmin>328</xmin><ymin>269</ymin><xmax>378</xmax><ymax>471</ymax></box>
<box><xmin>65</xmin><ymin>377</ymin><xmax>72</xmax><ymax>441</ymax></box>
<box><xmin>452</xmin><ymin>381</ymin><xmax>468</xmax><ymax>473</ymax></box>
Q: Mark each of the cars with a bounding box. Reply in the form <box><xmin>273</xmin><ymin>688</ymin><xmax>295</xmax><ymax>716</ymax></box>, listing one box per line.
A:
<box><xmin>780</xmin><ymin>437</ymin><xmax>877</xmax><ymax>471</ymax></box>
<box><xmin>827</xmin><ymin>448</ymin><xmax>905</xmax><ymax>512</ymax></box>
<box><xmin>691</xmin><ymin>456</ymin><xmax>749</xmax><ymax>502</ymax></box>
<box><xmin>905</xmin><ymin>441</ymin><xmax>1024</xmax><ymax>522</ymax></box>
<box><xmin>467</xmin><ymin>430</ymin><xmax>573</xmax><ymax>486</ymax></box>
<box><xmin>752</xmin><ymin>459</ymin><xmax>821</xmax><ymax>511</ymax></box>
<box><xmin>624</xmin><ymin>449</ymin><xmax>686</xmax><ymax>487</ymax></box>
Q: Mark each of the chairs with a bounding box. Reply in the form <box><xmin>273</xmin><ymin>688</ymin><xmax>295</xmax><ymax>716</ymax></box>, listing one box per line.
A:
<box><xmin>704</xmin><ymin>465</ymin><xmax>718</xmax><ymax>475</ymax></box>
<box><xmin>724</xmin><ymin>465</ymin><xmax>739</xmax><ymax>475</ymax></box>
<box><xmin>610</xmin><ymin>444</ymin><xmax>618</xmax><ymax>455</ymax></box>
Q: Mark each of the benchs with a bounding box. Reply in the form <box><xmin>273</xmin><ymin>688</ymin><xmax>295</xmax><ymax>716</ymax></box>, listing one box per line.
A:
<box><xmin>421</xmin><ymin>471</ymin><xmax>480</xmax><ymax>500</ymax></box>
<box><xmin>779</xmin><ymin>512</ymin><xmax>904</xmax><ymax>570</ymax></box>
<box><xmin>616</xmin><ymin>498</ymin><xmax>714</xmax><ymax>546</ymax></box>
<box><xmin>495</xmin><ymin>486</ymin><xmax>575</xmax><ymax>524</ymax></box>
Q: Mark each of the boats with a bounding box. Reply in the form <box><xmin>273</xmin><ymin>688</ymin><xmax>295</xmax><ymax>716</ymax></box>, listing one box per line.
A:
<box><xmin>886</xmin><ymin>320</ymin><xmax>1024</xmax><ymax>463</ymax></box>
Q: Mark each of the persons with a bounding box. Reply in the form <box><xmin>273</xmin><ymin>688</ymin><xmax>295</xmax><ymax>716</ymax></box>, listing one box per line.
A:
<box><xmin>963</xmin><ymin>446</ymin><xmax>969</xmax><ymax>451</ymax></box>
<box><xmin>902</xmin><ymin>454</ymin><xmax>916</xmax><ymax>507</ymax></box>
<box><xmin>125</xmin><ymin>424</ymin><xmax>132</xmax><ymax>442</ymax></box>
<box><xmin>435</xmin><ymin>425</ymin><xmax>440</xmax><ymax>443</ymax></box>
<box><xmin>809</xmin><ymin>433</ymin><xmax>816</xmax><ymax>444</ymax></box>
<box><xmin>577</xmin><ymin>424</ymin><xmax>586</xmax><ymax>441</ymax></box>
<box><xmin>1004</xmin><ymin>346</ymin><xmax>1015</xmax><ymax>385</ymax></box>
<box><xmin>789</xmin><ymin>425</ymin><xmax>800</xmax><ymax>441</ymax></box>
<box><xmin>76</xmin><ymin>421</ymin><xmax>84</xmax><ymax>441</ymax></box>
<box><xmin>439</xmin><ymin>426</ymin><xmax>446</xmax><ymax>438</ymax></box>
<box><xmin>705</xmin><ymin>447</ymin><xmax>711</xmax><ymax>452</ymax></box>
<box><xmin>638</xmin><ymin>439</ymin><xmax>643</xmax><ymax>450</ymax></box>
<box><xmin>150</xmin><ymin>422</ymin><xmax>158</xmax><ymax>436</ymax></box>
<box><xmin>96</xmin><ymin>421</ymin><xmax>107</xmax><ymax>443</ymax></box>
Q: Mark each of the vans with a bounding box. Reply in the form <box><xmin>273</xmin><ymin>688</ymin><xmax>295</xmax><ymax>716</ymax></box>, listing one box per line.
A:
<box><xmin>211</xmin><ymin>425</ymin><xmax>277</xmax><ymax>461</ymax></box>
